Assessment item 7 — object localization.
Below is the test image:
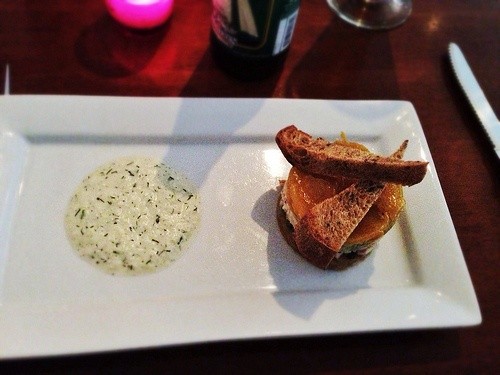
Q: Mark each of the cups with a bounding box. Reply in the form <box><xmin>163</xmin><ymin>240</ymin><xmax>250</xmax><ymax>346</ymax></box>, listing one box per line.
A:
<box><xmin>327</xmin><ymin>0</ymin><xmax>413</xmax><ymax>30</ymax></box>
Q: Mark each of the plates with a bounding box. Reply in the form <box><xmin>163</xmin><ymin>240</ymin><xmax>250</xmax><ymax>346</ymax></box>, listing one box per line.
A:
<box><xmin>0</xmin><ymin>94</ymin><xmax>482</xmax><ymax>360</ymax></box>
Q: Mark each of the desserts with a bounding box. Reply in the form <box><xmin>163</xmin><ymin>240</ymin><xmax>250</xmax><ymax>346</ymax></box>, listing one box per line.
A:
<box><xmin>275</xmin><ymin>125</ymin><xmax>429</xmax><ymax>273</ymax></box>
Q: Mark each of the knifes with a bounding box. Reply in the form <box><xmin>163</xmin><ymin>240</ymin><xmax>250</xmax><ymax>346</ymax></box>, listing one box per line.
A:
<box><xmin>449</xmin><ymin>43</ymin><xmax>500</xmax><ymax>161</ymax></box>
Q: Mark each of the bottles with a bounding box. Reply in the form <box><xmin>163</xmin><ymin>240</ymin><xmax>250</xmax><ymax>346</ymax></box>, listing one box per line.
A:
<box><xmin>105</xmin><ymin>0</ymin><xmax>174</xmax><ymax>31</ymax></box>
<box><xmin>209</xmin><ymin>0</ymin><xmax>301</xmax><ymax>59</ymax></box>
